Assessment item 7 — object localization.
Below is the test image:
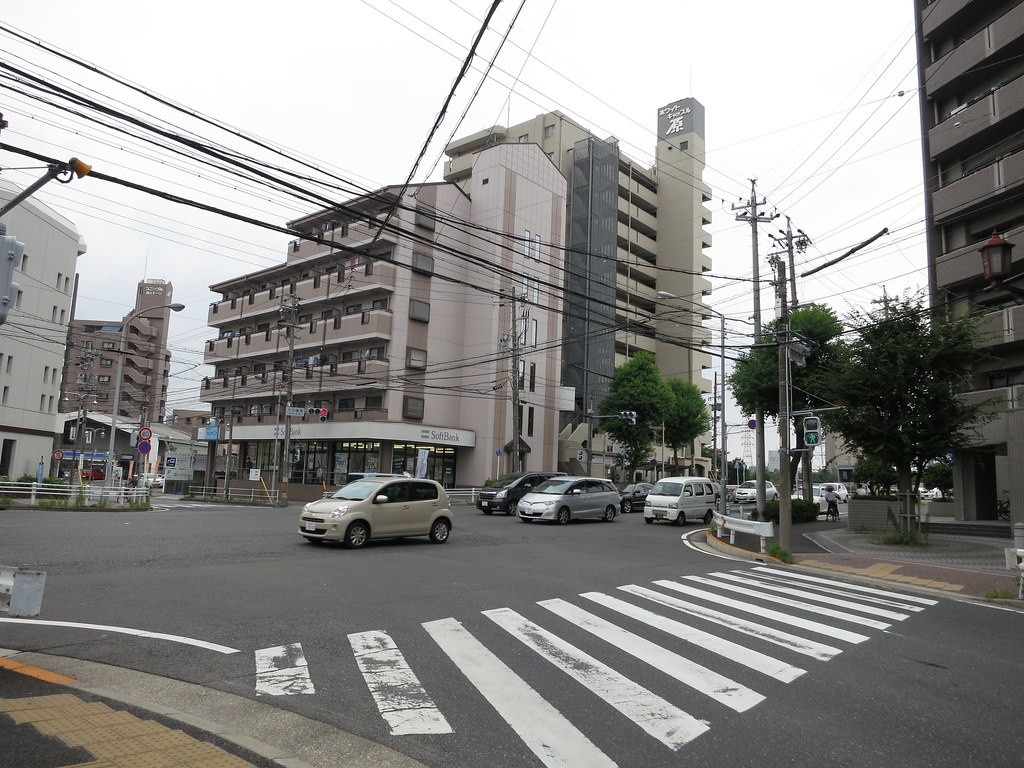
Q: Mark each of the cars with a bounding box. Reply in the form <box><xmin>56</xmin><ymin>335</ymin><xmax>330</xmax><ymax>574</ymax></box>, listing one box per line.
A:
<box><xmin>855</xmin><ymin>482</ymin><xmax>944</xmax><ymax>500</ymax></box>
<box><xmin>732</xmin><ymin>479</ymin><xmax>779</xmax><ymax>504</ymax></box>
<box><xmin>617</xmin><ymin>481</ymin><xmax>655</xmax><ymax>514</ymax></box>
<box><xmin>132</xmin><ymin>472</ymin><xmax>164</xmax><ymax>489</ymax></box>
<box><xmin>83</xmin><ymin>469</ymin><xmax>105</xmax><ymax>481</ymax></box>
<box><xmin>819</xmin><ymin>482</ymin><xmax>849</xmax><ymax>503</ymax></box>
<box><xmin>296</xmin><ymin>475</ymin><xmax>454</xmax><ymax>551</ymax></box>
<box><xmin>790</xmin><ymin>485</ymin><xmax>834</xmax><ymax>516</ymax></box>
<box><xmin>516</xmin><ymin>474</ymin><xmax>623</xmax><ymax>526</ymax></box>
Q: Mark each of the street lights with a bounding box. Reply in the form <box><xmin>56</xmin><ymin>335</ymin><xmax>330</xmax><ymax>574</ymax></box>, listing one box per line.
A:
<box><xmin>61</xmin><ymin>391</ymin><xmax>100</xmax><ymax>500</ymax></box>
<box><xmin>84</xmin><ymin>427</ymin><xmax>106</xmax><ymax>485</ymax></box>
<box><xmin>656</xmin><ymin>288</ymin><xmax>728</xmax><ymax>519</ymax></box>
<box><xmin>99</xmin><ymin>301</ymin><xmax>185</xmax><ymax>505</ymax></box>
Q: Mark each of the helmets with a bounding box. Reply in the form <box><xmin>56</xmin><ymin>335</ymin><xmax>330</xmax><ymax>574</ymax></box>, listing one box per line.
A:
<box><xmin>826</xmin><ymin>486</ymin><xmax>834</xmax><ymax>491</ymax></box>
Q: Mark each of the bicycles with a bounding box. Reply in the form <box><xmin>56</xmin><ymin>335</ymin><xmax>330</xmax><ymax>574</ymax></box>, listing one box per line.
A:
<box><xmin>829</xmin><ymin>506</ymin><xmax>837</xmax><ymax>522</ymax></box>
<box><xmin>116</xmin><ymin>488</ymin><xmax>134</xmax><ymax>504</ymax></box>
<box><xmin>996</xmin><ymin>489</ymin><xmax>1011</xmax><ymax>523</ymax></box>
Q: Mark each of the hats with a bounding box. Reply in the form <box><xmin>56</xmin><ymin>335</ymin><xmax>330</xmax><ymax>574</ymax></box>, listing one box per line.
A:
<box><xmin>133</xmin><ymin>475</ymin><xmax>138</xmax><ymax>478</ymax></box>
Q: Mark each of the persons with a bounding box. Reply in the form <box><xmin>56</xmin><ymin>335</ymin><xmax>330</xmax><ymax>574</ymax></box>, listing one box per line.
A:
<box><xmin>824</xmin><ymin>485</ymin><xmax>841</xmax><ymax>521</ymax></box>
<box><xmin>246</xmin><ymin>457</ymin><xmax>253</xmax><ymax>473</ymax></box>
<box><xmin>853</xmin><ymin>484</ymin><xmax>866</xmax><ymax>496</ymax></box>
<box><xmin>127</xmin><ymin>474</ymin><xmax>138</xmax><ymax>491</ymax></box>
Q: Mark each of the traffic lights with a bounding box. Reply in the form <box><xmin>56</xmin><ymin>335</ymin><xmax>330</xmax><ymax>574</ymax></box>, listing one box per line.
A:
<box><xmin>307</xmin><ymin>407</ymin><xmax>328</xmax><ymax>423</ymax></box>
<box><xmin>617</xmin><ymin>411</ymin><xmax>637</xmax><ymax>426</ymax></box>
<box><xmin>802</xmin><ymin>416</ymin><xmax>822</xmax><ymax>447</ymax></box>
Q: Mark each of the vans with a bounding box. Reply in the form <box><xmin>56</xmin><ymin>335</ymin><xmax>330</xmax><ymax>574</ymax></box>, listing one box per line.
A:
<box><xmin>643</xmin><ymin>476</ymin><xmax>716</xmax><ymax>527</ymax></box>
<box><xmin>474</xmin><ymin>470</ymin><xmax>570</xmax><ymax>517</ymax></box>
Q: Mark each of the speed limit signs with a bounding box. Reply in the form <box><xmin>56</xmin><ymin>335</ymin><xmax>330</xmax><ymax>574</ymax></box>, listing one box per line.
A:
<box><xmin>139</xmin><ymin>427</ymin><xmax>152</xmax><ymax>441</ymax></box>
